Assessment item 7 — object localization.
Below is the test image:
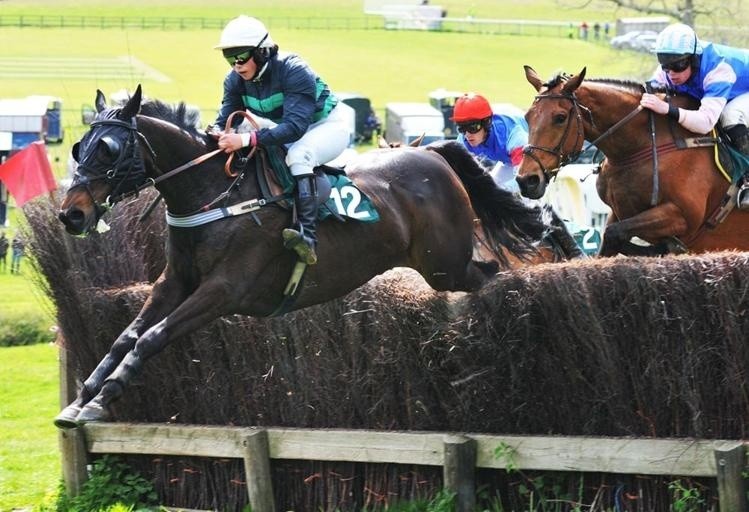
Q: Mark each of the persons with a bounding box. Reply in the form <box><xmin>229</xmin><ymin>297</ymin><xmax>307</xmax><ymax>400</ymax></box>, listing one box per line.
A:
<box><xmin>10</xmin><ymin>232</ymin><xmax>25</xmax><ymax>275</ymax></box>
<box><xmin>0</xmin><ymin>231</ymin><xmax>9</xmax><ymax>271</ymax></box>
<box><xmin>640</xmin><ymin>23</ymin><xmax>749</xmax><ymax>210</ymax></box>
<box><xmin>448</xmin><ymin>94</ymin><xmax>529</xmax><ymax>194</ymax></box>
<box><xmin>206</xmin><ymin>15</ymin><xmax>354</xmax><ymax>265</ymax></box>
<box><xmin>580</xmin><ymin>22</ymin><xmax>609</xmax><ymax>43</ymax></box>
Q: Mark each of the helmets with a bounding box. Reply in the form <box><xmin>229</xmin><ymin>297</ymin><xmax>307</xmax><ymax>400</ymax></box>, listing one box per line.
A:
<box><xmin>213</xmin><ymin>15</ymin><xmax>274</xmax><ymax>48</ymax></box>
<box><xmin>654</xmin><ymin>22</ymin><xmax>702</xmax><ymax>55</ymax></box>
<box><xmin>448</xmin><ymin>93</ymin><xmax>492</xmax><ymax>123</ymax></box>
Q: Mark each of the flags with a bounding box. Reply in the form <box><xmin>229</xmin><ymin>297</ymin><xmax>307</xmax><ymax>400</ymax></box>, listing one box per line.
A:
<box><xmin>0</xmin><ymin>141</ymin><xmax>58</xmax><ymax>208</ymax></box>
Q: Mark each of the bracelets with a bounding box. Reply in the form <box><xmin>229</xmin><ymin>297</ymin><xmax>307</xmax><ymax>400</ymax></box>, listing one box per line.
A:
<box><xmin>250</xmin><ymin>131</ymin><xmax>257</xmax><ymax>147</ymax></box>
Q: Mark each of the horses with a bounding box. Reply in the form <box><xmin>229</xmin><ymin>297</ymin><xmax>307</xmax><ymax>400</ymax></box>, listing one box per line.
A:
<box><xmin>374</xmin><ymin>131</ymin><xmax>559</xmax><ymax>272</ymax></box>
<box><xmin>53</xmin><ymin>84</ymin><xmax>562</xmax><ymax>430</ymax></box>
<box><xmin>515</xmin><ymin>64</ymin><xmax>749</xmax><ymax>260</ymax></box>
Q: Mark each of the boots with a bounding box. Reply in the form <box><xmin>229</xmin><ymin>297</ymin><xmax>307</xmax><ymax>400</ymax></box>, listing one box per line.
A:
<box><xmin>281</xmin><ymin>196</ymin><xmax>319</xmax><ymax>265</ymax></box>
<box><xmin>737</xmin><ymin>139</ymin><xmax>749</xmax><ymax>207</ymax></box>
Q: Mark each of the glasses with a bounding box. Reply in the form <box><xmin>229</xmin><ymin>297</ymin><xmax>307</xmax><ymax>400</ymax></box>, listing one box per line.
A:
<box><xmin>458</xmin><ymin>119</ymin><xmax>486</xmax><ymax>134</ymax></box>
<box><xmin>222</xmin><ymin>48</ymin><xmax>259</xmax><ymax>67</ymax></box>
<box><xmin>655</xmin><ymin>56</ymin><xmax>692</xmax><ymax>73</ymax></box>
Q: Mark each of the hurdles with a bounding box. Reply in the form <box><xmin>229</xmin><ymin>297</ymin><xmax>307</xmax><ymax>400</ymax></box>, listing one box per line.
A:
<box><xmin>59</xmin><ymin>331</ymin><xmax>749</xmax><ymax>510</ymax></box>
